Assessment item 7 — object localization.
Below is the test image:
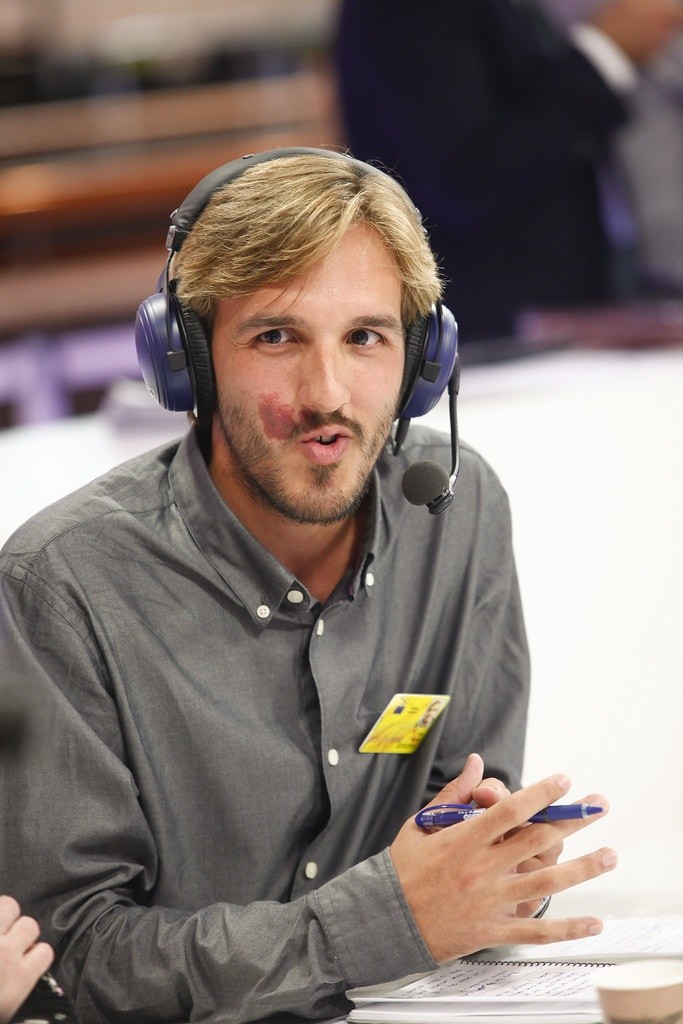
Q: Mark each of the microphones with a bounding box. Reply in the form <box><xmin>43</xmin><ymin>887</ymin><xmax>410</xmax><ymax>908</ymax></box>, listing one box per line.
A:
<box><xmin>401</xmin><ymin>351</ymin><xmax>460</xmax><ymax>515</ymax></box>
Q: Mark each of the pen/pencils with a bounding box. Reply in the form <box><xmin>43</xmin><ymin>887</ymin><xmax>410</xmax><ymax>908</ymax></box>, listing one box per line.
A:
<box><xmin>415</xmin><ymin>803</ymin><xmax>604</xmax><ymax>832</ymax></box>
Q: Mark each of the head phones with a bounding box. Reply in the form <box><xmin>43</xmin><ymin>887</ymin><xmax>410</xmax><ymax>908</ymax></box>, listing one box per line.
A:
<box><xmin>135</xmin><ymin>146</ymin><xmax>459</xmax><ymax>455</ymax></box>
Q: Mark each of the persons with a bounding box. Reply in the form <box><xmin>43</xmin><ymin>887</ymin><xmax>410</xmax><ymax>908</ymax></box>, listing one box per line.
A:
<box><xmin>0</xmin><ymin>149</ymin><xmax>620</xmax><ymax>1024</ymax></box>
<box><xmin>333</xmin><ymin>0</ymin><xmax>681</xmax><ymax>361</ymax></box>
<box><xmin>0</xmin><ymin>895</ymin><xmax>54</xmax><ymax>1024</ymax></box>
